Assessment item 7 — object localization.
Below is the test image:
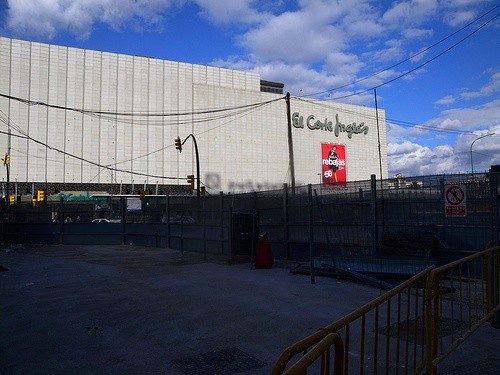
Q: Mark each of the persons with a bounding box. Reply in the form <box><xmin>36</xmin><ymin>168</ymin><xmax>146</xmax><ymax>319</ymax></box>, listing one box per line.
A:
<box><xmin>327</xmin><ymin>146</ymin><xmax>338</xmax><ymax>185</ymax></box>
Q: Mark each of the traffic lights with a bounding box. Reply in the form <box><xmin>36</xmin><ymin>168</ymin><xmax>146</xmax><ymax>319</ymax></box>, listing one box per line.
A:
<box><xmin>4</xmin><ymin>153</ymin><xmax>10</xmax><ymax>167</ymax></box>
<box><xmin>175</xmin><ymin>135</ymin><xmax>183</xmax><ymax>153</ymax></box>
<box><xmin>36</xmin><ymin>191</ymin><xmax>45</xmax><ymax>202</ymax></box>
<box><xmin>187</xmin><ymin>175</ymin><xmax>194</xmax><ymax>190</ymax></box>
<box><xmin>9</xmin><ymin>196</ymin><xmax>14</xmax><ymax>205</ymax></box>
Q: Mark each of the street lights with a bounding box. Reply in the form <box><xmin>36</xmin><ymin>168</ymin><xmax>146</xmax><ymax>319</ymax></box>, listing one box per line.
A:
<box><xmin>470</xmin><ymin>131</ymin><xmax>495</xmax><ymax>177</ymax></box>
